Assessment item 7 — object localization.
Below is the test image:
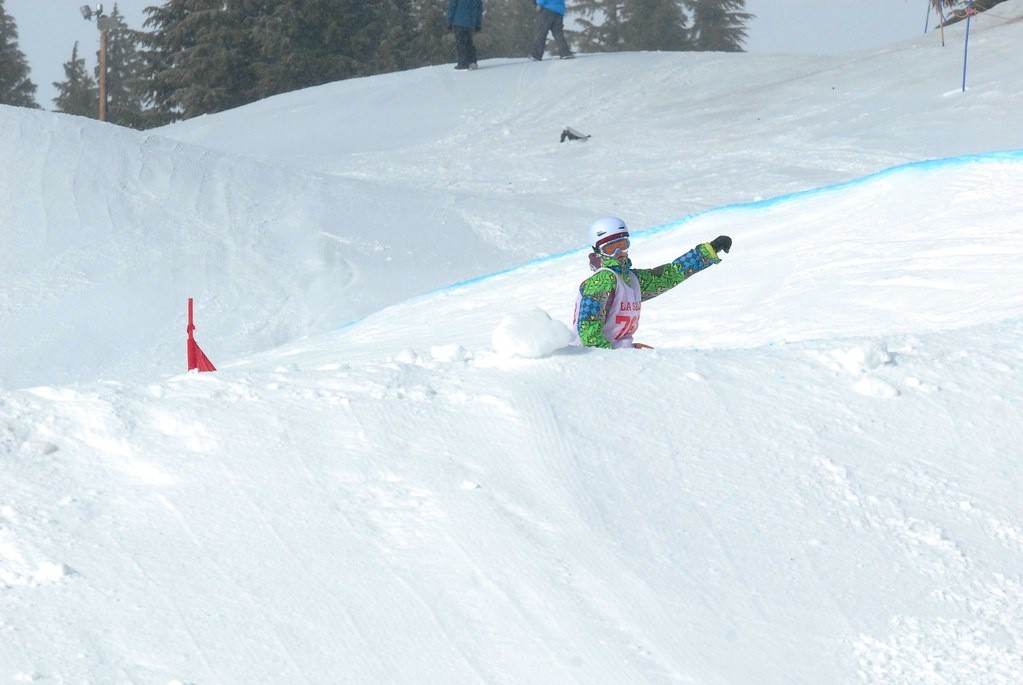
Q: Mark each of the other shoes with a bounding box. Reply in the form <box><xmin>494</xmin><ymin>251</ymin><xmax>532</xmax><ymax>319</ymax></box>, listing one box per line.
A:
<box><xmin>454</xmin><ymin>62</ymin><xmax>469</xmax><ymax>69</ymax></box>
<box><xmin>560</xmin><ymin>52</ymin><xmax>573</xmax><ymax>58</ymax></box>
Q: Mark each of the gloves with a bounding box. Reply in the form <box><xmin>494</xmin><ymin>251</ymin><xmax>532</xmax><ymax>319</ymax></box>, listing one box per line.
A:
<box><xmin>710</xmin><ymin>235</ymin><xmax>733</xmax><ymax>253</ymax></box>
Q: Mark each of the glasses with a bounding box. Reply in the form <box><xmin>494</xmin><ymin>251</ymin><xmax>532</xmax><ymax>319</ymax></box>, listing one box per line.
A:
<box><xmin>598</xmin><ymin>236</ymin><xmax>631</xmax><ymax>258</ymax></box>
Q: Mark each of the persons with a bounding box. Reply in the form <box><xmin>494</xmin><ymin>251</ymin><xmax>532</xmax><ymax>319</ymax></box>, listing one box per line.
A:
<box><xmin>530</xmin><ymin>1</ymin><xmax>573</xmax><ymax>62</ymax></box>
<box><xmin>571</xmin><ymin>216</ymin><xmax>732</xmax><ymax>350</ymax></box>
<box><xmin>446</xmin><ymin>0</ymin><xmax>484</xmax><ymax>71</ymax></box>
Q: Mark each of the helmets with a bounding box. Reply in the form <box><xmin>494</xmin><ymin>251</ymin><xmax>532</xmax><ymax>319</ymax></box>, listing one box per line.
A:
<box><xmin>590</xmin><ymin>216</ymin><xmax>629</xmax><ymax>248</ymax></box>
